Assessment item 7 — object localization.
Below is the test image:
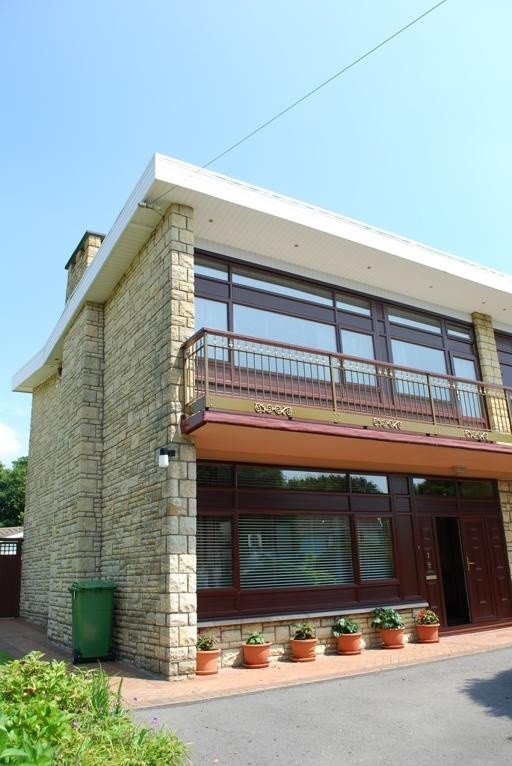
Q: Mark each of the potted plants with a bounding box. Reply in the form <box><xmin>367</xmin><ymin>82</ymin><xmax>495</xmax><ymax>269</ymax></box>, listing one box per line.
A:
<box><xmin>334</xmin><ymin>616</ymin><xmax>362</xmax><ymax>655</ymax></box>
<box><xmin>372</xmin><ymin>608</ymin><xmax>406</xmax><ymax>650</ymax></box>
<box><xmin>240</xmin><ymin>632</ymin><xmax>271</xmax><ymax>669</ymax></box>
<box><xmin>290</xmin><ymin>622</ymin><xmax>318</xmax><ymax>661</ymax></box>
<box><xmin>196</xmin><ymin>634</ymin><xmax>220</xmax><ymax>675</ymax></box>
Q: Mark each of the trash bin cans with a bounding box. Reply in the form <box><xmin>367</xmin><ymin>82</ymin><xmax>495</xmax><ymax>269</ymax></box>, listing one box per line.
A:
<box><xmin>68</xmin><ymin>580</ymin><xmax>117</xmax><ymax>665</ymax></box>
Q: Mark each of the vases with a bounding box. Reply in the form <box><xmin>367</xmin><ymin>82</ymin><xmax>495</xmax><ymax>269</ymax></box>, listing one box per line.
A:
<box><xmin>415</xmin><ymin>624</ymin><xmax>439</xmax><ymax>644</ymax></box>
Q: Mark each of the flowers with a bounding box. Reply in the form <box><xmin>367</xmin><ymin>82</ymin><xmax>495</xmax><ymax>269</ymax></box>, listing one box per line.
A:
<box><xmin>416</xmin><ymin>608</ymin><xmax>438</xmax><ymax>623</ymax></box>
